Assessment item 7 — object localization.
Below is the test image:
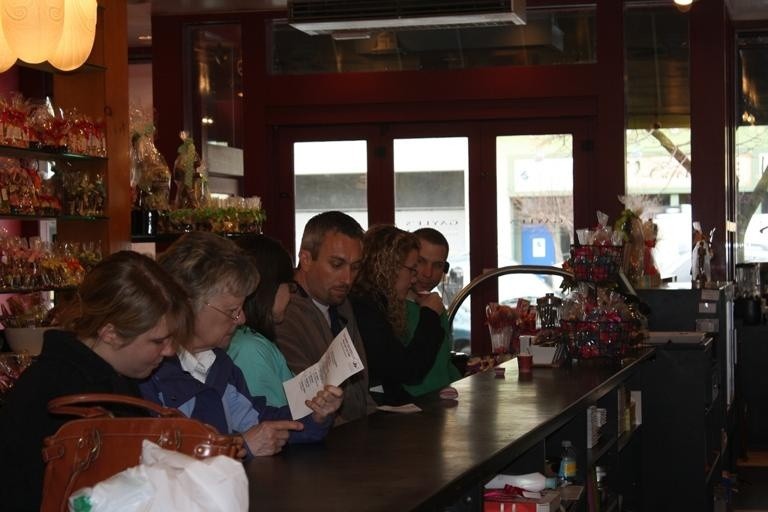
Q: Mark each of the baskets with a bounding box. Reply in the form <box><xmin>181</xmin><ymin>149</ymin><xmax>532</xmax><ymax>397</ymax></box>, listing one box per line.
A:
<box><xmin>560</xmin><ymin>320</ymin><xmax>633</xmax><ymax>358</ymax></box>
<box><xmin>570</xmin><ymin>243</ymin><xmax>624</xmax><ymax>284</ymax></box>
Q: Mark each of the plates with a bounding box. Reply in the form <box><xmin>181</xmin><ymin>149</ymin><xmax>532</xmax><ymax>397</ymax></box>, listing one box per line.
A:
<box><xmin>587</xmin><ymin>406</ymin><xmax>607</xmax><ymax>450</ymax></box>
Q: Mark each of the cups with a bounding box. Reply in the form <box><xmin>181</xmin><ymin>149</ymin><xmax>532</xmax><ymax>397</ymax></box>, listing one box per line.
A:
<box><xmin>493</xmin><ymin>367</ymin><xmax>505</xmax><ymax>377</ymax></box>
<box><xmin>516</xmin><ymin>354</ymin><xmax>532</xmax><ymax>373</ymax></box>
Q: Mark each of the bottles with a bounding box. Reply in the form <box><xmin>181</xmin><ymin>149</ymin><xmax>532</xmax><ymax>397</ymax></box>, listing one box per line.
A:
<box><xmin>559</xmin><ymin>440</ymin><xmax>577</xmax><ymax>484</ymax></box>
<box><xmin>143</xmin><ymin>209</ymin><xmax>159</xmax><ymax>237</ymax></box>
<box><xmin>536</xmin><ymin>292</ymin><xmax>562</xmax><ymax>327</ymax></box>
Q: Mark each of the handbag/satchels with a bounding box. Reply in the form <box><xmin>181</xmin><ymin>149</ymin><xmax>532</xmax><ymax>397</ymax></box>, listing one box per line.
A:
<box><xmin>39</xmin><ymin>393</ymin><xmax>248</xmax><ymax>512</ymax></box>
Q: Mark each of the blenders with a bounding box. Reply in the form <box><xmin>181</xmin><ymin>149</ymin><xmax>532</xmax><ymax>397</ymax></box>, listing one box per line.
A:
<box><xmin>733</xmin><ymin>262</ymin><xmax>761</xmax><ymax>326</ymax></box>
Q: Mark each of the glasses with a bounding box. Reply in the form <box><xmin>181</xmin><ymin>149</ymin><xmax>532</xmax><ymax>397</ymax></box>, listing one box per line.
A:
<box><xmin>191</xmin><ymin>296</ymin><xmax>242</xmax><ymax>320</ymax></box>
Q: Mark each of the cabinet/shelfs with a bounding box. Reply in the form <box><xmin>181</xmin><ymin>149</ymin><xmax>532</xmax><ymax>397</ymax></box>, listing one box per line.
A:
<box><xmin>421</xmin><ymin>364</ymin><xmax>645</xmax><ymax>512</ymax></box>
<box><xmin>0</xmin><ymin>90</ymin><xmax>108</xmax><ymax>394</ymax></box>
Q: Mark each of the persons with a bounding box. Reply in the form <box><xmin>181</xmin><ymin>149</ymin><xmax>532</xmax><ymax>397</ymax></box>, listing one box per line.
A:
<box><xmin>0</xmin><ymin>212</ymin><xmax>464</xmax><ymax>512</ymax></box>
